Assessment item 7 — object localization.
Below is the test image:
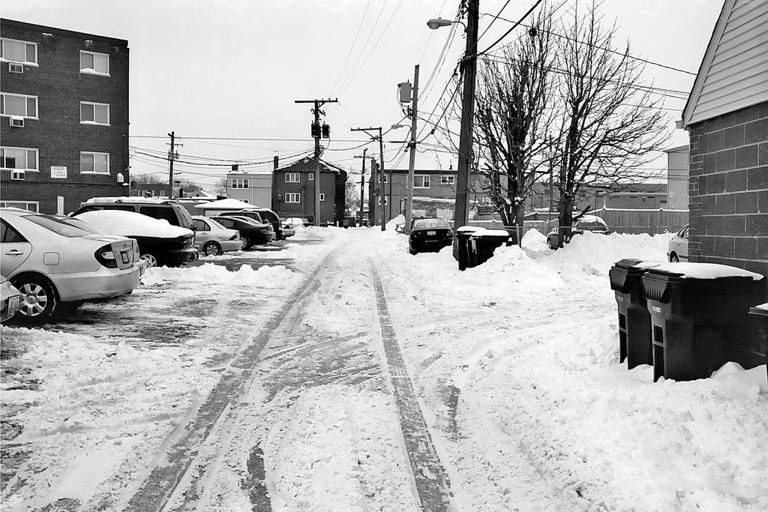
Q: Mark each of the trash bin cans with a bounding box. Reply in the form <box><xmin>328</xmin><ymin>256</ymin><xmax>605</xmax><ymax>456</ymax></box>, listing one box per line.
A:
<box><xmin>457</xmin><ymin>231</ymin><xmax>509</xmax><ymax>271</ymax></box>
<box><xmin>609</xmin><ymin>259</ymin><xmax>764</xmax><ymax>383</ymax></box>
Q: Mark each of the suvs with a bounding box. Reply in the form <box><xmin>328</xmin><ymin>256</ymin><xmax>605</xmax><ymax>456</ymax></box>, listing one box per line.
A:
<box><xmin>546</xmin><ymin>214</ymin><xmax>610</xmax><ymax>250</ymax></box>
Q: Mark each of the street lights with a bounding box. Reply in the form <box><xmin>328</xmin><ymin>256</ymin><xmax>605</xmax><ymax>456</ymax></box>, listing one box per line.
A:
<box><xmin>390</xmin><ymin>123</ymin><xmax>417</xmax><ymax>235</ymax></box>
<box><xmin>426</xmin><ymin>18</ymin><xmax>479</xmax><ymax>262</ymax></box>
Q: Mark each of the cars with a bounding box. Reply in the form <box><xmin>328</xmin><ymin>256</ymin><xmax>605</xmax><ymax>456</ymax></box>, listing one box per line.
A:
<box><xmin>667</xmin><ymin>223</ymin><xmax>688</xmax><ymax>263</ymax></box>
<box><xmin>408</xmin><ymin>217</ymin><xmax>454</xmax><ymax>255</ymax></box>
